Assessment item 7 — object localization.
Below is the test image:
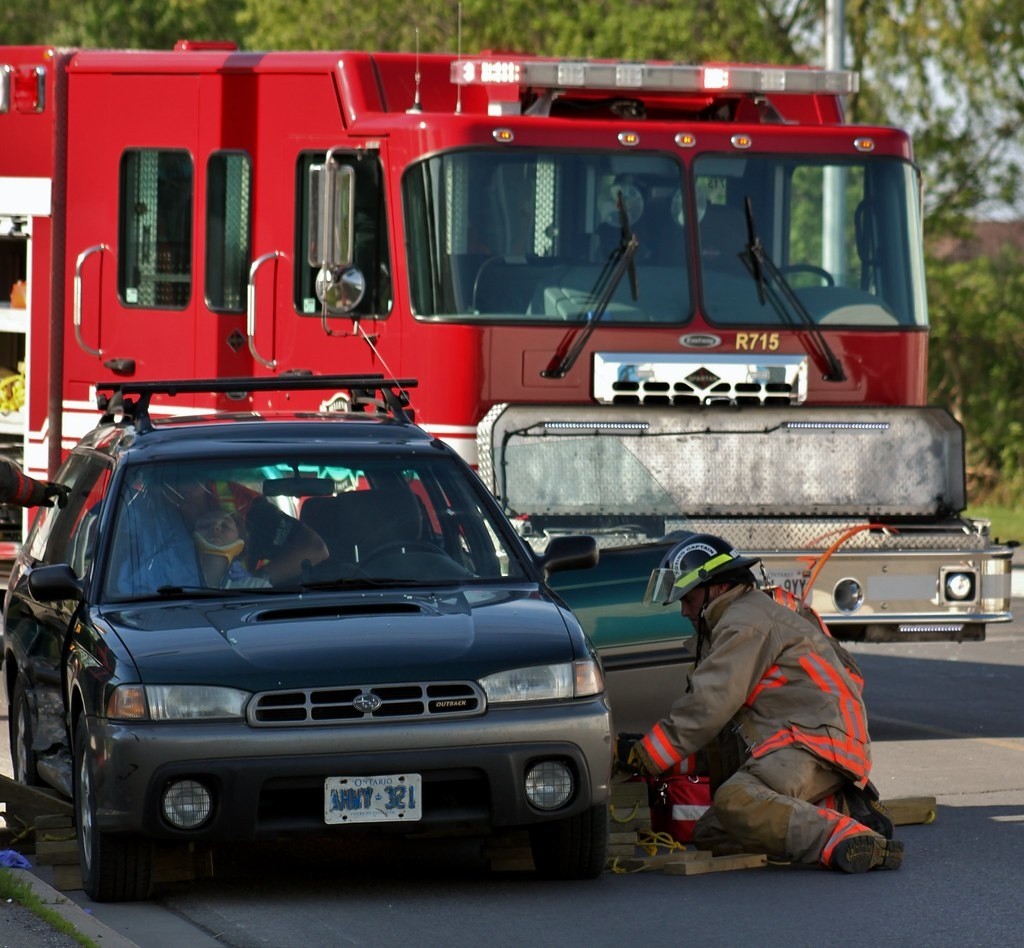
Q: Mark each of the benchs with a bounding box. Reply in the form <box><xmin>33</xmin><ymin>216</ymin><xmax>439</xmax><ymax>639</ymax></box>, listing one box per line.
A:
<box><xmin>470</xmin><ymin>257</ymin><xmax>770</xmax><ymax>325</ymax></box>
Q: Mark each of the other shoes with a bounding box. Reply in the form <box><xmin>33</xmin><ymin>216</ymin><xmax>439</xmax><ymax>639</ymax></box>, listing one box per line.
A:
<box><xmin>842</xmin><ymin>775</ymin><xmax>895</xmax><ymax>840</ymax></box>
<box><xmin>830</xmin><ymin>830</ymin><xmax>903</xmax><ymax>874</ymax></box>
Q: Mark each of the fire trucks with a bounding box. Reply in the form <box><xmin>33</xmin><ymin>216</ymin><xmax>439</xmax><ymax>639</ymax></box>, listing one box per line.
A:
<box><xmin>0</xmin><ymin>38</ymin><xmax>1013</xmax><ymax>637</ymax></box>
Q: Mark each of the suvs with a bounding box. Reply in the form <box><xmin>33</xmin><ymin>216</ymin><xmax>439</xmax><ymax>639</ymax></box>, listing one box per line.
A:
<box><xmin>1</xmin><ymin>375</ymin><xmax>611</xmax><ymax>904</ymax></box>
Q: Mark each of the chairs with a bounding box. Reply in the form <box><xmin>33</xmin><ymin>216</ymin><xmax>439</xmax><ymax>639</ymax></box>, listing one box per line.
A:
<box><xmin>67</xmin><ymin>489</ymin><xmax>477</xmax><ymax>597</ymax></box>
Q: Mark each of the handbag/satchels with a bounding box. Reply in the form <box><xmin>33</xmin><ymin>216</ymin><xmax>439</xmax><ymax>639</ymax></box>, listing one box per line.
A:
<box><xmin>640</xmin><ymin>757</ymin><xmax>712</xmax><ymax>843</ymax></box>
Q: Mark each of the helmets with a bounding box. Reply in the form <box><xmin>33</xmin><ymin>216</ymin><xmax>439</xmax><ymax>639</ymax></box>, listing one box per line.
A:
<box><xmin>657</xmin><ymin>534</ymin><xmax>762</xmax><ymax>608</ymax></box>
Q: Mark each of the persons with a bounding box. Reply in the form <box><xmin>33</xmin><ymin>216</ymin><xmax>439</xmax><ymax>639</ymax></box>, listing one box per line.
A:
<box><xmin>120</xmin><ymin>495</ymin><xmax>329</xmax><ymax>598</ymax></box>
<box><xmin>623</xmin><ymin>535</ymin><xmax>906</xmax><ymax>872</ymax></box>
<box><xmin>1</xmin><ymin>458</ymin><xmax>75</xmax><ymax>509</ymax></box>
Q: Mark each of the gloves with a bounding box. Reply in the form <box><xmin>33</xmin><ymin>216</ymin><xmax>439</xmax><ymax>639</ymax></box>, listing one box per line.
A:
<box><xmin>35</xmin><ymin>481</ymin><xmax>72</xmax><ymax>509</ymax></box>
